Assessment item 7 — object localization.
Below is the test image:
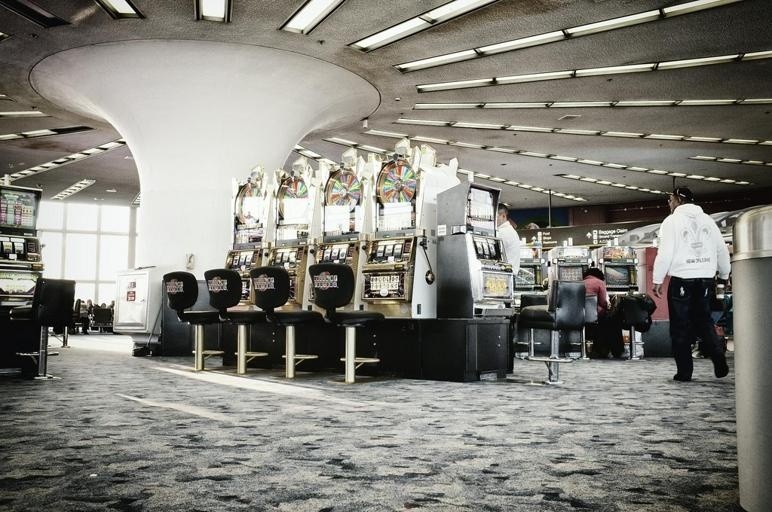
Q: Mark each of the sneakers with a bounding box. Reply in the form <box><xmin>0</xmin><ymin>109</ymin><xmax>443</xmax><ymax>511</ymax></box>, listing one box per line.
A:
<box><xmin>674</xmin><ymin>374</ymin><xmax>691</xmax><ymax>381</ymax></box>
<box><xmin>713</xmin><ymin>351</ymin><xmax>728</xmax><ymax>377</ymax></box>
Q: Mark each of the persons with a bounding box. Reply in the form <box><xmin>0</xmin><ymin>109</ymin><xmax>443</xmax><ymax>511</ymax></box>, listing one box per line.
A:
<box><xmin>75</xmin><ymin>297</ymin><xmax>114</xmax><ymax>334</ymax></box>
<box><xmin>580</xmin><ymin>268</ymin><xmax>630</xmax><ymax>360</ymax></box>
<box><xmin>496</xmin><ymin>202</ymin><xmax>522</xmax><ymax>374</ymax></box>
<box><xmin>650</xmin><ymin>185</ymin><xmax>731</xmax><ymax>382</ymax></box>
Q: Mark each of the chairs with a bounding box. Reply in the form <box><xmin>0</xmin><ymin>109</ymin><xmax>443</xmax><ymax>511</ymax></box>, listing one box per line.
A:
<box><xmin>0</xmin><ymin>276</ymin><xmax>114</xmax><ymax>382</ymax></box>
<box><xmin>163</xmin><ymin>263</ymin><xmax>385</xmax><ymax>385</ymax></box>
<box><xmin>518</xmin><ymin>280</ymin><xmax>657</xmax><ymax>384</ymax></box>
